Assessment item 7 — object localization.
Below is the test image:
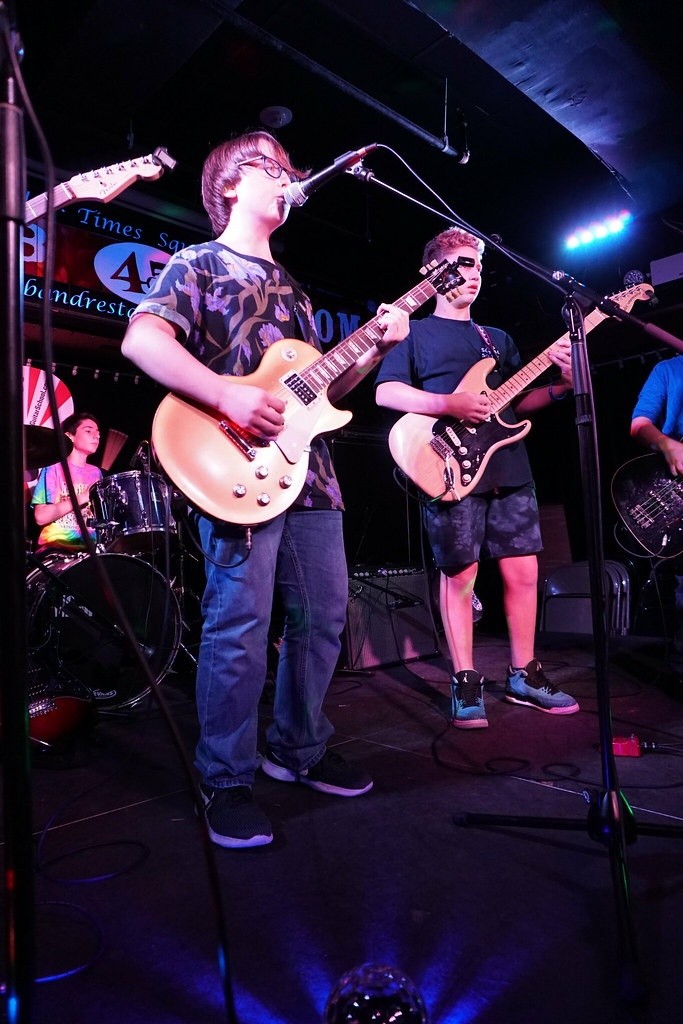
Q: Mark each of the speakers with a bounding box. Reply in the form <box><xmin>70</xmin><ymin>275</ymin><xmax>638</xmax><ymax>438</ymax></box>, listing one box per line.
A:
<box><xmin>334</xmin><ymin>566</ymin><xmax>439</xmax><ymax>671</ymax></box>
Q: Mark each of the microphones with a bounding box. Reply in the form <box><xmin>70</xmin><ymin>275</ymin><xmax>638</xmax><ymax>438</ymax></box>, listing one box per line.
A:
<box><xmin>283</xmin><ymin>144</ymin><xmax>380</xmax><ymax>208</ymax></box>
<box><xmin>137</xmin><ymin>642</ymin><xmax>155</xmax><ymax>658</ymax></box>
<box><xmin>389</xmin><ymin>601</ymin><xmax>421</xmax><ymax>610</ymax></box>
<box><xmin>129</xmin><ymin>441</ymin><xmax>146</xmax><ymax>468</ymax></box>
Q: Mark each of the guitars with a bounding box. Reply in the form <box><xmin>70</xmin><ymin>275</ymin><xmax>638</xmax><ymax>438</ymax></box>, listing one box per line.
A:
<box><xmin>25</xmin><ymin>145</ymin><xmax>177</xmax><ymax>228</ymax></box>
<box><xmin>387</xmin><ymin>283</ymin><xmax>659</xmax><ymax>505</ymax></box>
<box><xmin>148</xmin><ymin>255</ymin><xmax>478</xmax><ymax>529</ymax></box>
<box><xmin>609</xmin><ymin>437</ymin><xmax>683</xmax><ymax>561</ymax></box>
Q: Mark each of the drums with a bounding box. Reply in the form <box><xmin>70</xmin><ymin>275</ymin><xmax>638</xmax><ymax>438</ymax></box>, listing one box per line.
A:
<box><xmin>25</xmin><ymin>551</ymin><xmax>184</xmax><ymax>712</ymax></box>
<box><xmin>89</xmin><ymin>470</ymin><xmax>177</xmax><ymax>556</ymax></box>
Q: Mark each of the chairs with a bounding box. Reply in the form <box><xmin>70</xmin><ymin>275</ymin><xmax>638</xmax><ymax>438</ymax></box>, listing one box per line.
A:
<box><xmin>539</xmin><ymin>560</ymin><xmax>631</xmax><ymax>634</ymax></box>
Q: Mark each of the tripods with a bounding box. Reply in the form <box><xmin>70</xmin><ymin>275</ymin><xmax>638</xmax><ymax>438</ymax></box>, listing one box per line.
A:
<box><xmin>344</xmin><ymin>164</ymin><xmax>683</xmax><ymax>1024</ymax></box>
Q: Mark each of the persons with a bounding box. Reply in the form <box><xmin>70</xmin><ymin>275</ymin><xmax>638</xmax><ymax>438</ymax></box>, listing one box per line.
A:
<box><xmin>29</xmin><ymin>414</ymin><xmax>104</xmax><ymax>558</ymax></box>
<box><xmin>119</xmin><ymin>130</ymin><xmax>410</xmax><ymax>849</ymax></box>
<box><xmin>630</xmin><ymin>354</ymin><xmax>683</xmax><ymax>699</ymax></box>
<box><xmin>374</xmin><ymin>228</ymin><xmax>581</xmax><ymax>728</ymax></box>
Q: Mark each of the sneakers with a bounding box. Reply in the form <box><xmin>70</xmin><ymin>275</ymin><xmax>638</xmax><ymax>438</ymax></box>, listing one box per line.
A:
<box><xmin>262</xmin><ymin>747</ymin><xmax>372</xmax><ymax>797</ymax></box>
<box><xmin>449</xmin><ymin>669</ymin><xmax>489</xmax><ymax>729</ymax></box>
<box><xmin>504</xmin><ymin>659</ymin><xmax>580</xmax><ymax>714</ymax></box>
<box><xmin>194</xmin><ymin>781</ymin><xmax>272</xmax><ymax>848</ymax></box>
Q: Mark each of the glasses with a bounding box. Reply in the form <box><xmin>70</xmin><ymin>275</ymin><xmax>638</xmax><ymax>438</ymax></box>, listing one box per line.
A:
<box><xmin>236</xmin><ymin>155</ymin><xmax>298</xmax><ymax>184</ymax></box>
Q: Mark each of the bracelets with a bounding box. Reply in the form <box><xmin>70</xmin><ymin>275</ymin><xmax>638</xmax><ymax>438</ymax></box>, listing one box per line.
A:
<box><xmin>549</xmin><ymin>382</ymin><xmax>567</xmax><ymax>401</ymax></box>
